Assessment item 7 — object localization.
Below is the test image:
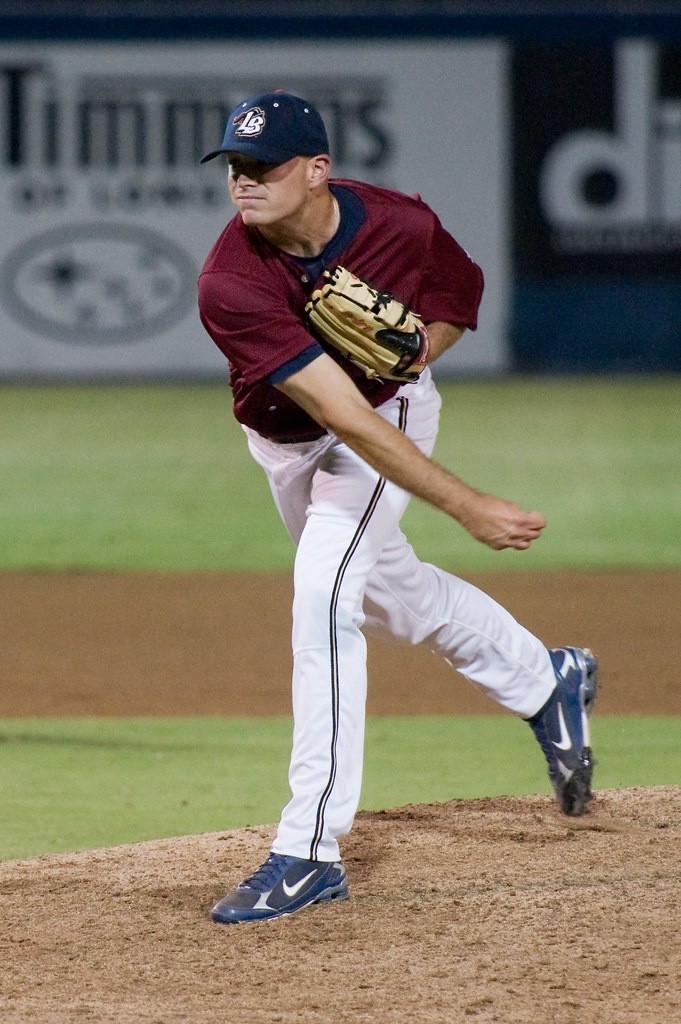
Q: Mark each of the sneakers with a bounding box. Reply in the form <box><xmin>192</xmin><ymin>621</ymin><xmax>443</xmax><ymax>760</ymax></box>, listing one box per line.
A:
<box><xmin>211</xmin><ymin>852</ymin><xmax>350</xmax><ymax>925</ymax></box>
<box><xmin>529</xmin><ymin>646</ymin><xmax>598</xmax><ymax>817</ymax></box>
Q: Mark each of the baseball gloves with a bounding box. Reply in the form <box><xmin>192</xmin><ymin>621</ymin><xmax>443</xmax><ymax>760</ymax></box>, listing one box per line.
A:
<box><xmin>305</xmin><ymin>266</ymin><xmax>429</xmax><ymax>387</ymax></box>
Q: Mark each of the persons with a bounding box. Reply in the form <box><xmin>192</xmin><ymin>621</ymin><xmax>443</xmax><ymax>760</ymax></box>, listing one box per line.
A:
<box><xmin>198</xmin><ymin>95</ymin><xmax>597</xmax><ymax>924</ymax></box>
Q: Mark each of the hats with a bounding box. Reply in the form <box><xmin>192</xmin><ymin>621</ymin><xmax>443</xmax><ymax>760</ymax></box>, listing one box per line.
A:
<box><xmin>199</xmin><ymin>89</ymin><xmax>329</xmax><ymax>166</ymax></box>
